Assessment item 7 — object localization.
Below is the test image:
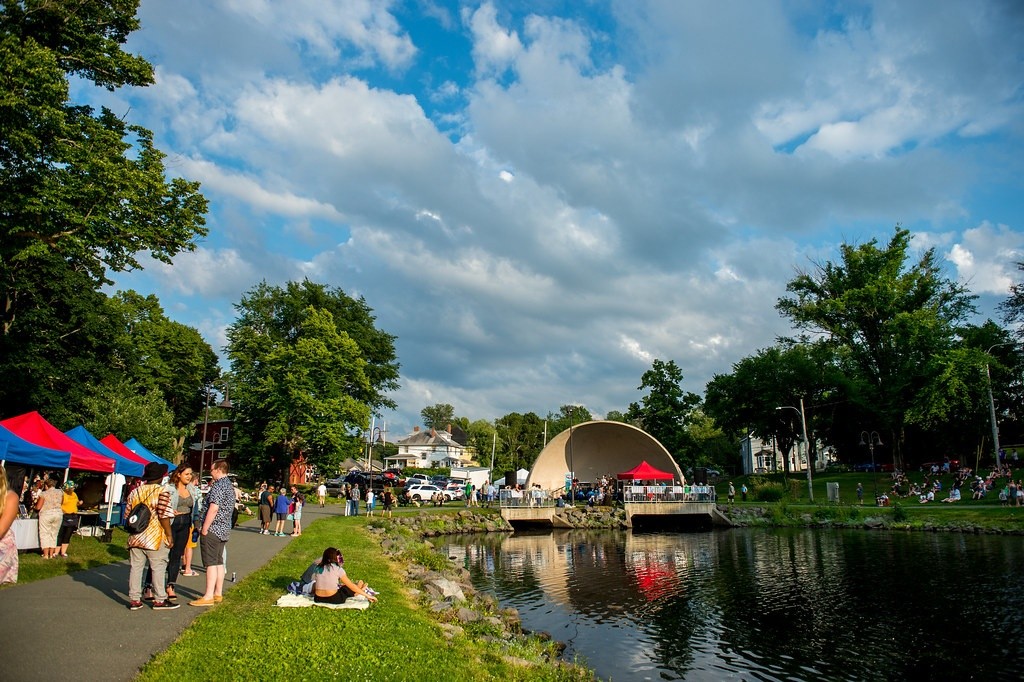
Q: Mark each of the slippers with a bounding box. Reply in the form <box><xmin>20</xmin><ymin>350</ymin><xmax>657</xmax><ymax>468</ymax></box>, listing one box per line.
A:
<box><xmin>183</xmin><ymin>570</ymin><xmax>201</xmax><ymax>576</ymax></box>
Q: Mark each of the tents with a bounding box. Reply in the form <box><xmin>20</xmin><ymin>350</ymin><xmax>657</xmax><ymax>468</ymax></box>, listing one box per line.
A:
<box><xmin>616</xmin><ymin>460</ymin><xmax>675</xmax><ymax>503</ymax></box>
<box><xmin>0</xmin><ymin>411</ymin><xmax>179</xmax><ymax>530</ymax></box>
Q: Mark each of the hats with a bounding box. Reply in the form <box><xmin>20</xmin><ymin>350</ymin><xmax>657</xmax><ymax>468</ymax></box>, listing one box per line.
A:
<box><xmin>141</xmin><ymin>462</ymin><xmax>168</xmax><ymax>480</ymax></box>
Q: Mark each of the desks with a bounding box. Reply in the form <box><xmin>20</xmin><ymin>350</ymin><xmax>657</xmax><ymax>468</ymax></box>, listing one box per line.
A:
<box><xmin>11</xmin><ymin>518</ymin><xmax>41</xmax><ymax>549</ymax></box>
<box><xmin>77</xmin><ymin>512</ymin><xmax>99</xmax><ymax>539</ymax></box>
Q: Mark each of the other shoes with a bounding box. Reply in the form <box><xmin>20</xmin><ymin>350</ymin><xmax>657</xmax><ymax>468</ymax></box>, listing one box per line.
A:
<box><xmin>62</xmin><ymin>553</ymin><xmax>68</xmax><ymax>558</ymax></box>
<box><xmin>291</xmin><ymin>532</ymin><xmax>300</xmax><ymax>537</ymax></box>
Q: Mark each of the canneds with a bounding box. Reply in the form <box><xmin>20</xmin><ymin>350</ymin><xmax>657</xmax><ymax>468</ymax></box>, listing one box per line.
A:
<box><xmin>232</xmin><ymin>572</ymin><xmax>236</xmax><ymax>584</ymax></box>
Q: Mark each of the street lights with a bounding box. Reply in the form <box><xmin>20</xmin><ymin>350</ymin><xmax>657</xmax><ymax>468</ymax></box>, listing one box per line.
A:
<box><xmin>986</xmin><ymin>342</ymin><xmax>1006</xmax><ymax>470</ymax></box>
<box><xmin>860</xmin><ymin>430</ymin><xmax>884</xmax><ymax>503</ymax></box>
<box><xmin>362</xmin><ymin>427</ymin><xmax>385</xmax><ymax>489</ymax></box>
<box><xmin>774</xmin><ymin>399</ymin><xmax>815</xmax><ymax>501</ymax></box>
<box><xmin>198</xmin><ymin>381</ymin><xmax>234</xmax><ymax>487</ymax></box>
<box><xmin>566</xmin><ymin>404</ymin><xmax>579</xmax><ymax>507</ymax></box>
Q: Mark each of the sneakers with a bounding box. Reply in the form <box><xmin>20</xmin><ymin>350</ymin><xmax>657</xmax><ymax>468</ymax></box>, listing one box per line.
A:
<box><xmin>129</xmin><ymin>601</ymin><xmax>145</xmax><ymax>611</ymax></box>
<box><xmin>259</xmin><ymin>528</ymin><xmax>271</xmax><ymax>535</ymax></box>
<box><xmin>190</xmin><ymin>594</ymin><xmax>223</xmax><ymax>606</ymax></box>
<box><xmin>274</xmin><ymin>531</ymin><xmax>287</xmax><ymax>537</ymax></box>
<box><xmin>153</xmin><ymin>599</ymin><xmax>181</xmax><ymax>609</ymax></box>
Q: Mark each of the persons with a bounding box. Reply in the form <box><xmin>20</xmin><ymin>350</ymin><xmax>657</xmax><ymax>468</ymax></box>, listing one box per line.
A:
<box><xmin>466</xmin><ymin>480</ymin><xmax>543</xmax><ymax>508</ymax></box>
<box><xmin>122</xmin><ymin>460</ymin><xmax>254</xmax><ymax>608</ymax></box>
<box><xmin>856</xmin><ymin>482</ymin><xmax>864</xmax><ymax>504</ymax></box>
<box><xmin>556</xmin><ymin>472</ymin><xmax>719</xmax><ymax>507</ymax></box>
<box><xmin>0</xmin><ymin>466</ymin><xmax>19</xmax><ymax>584</ymax></box>
<box><xmin>20</xmin><ymin>472</ymin><xmax>84</xmax><ymax>558</ymax></box>
<box><xmin>258</xmin><ymin>484</ymin><xmax>303</xmax><ymax>537</ymax></box>
<box><xmin>405</xmin><ymin>490</ymin><xmax>420</xmax><ymax>508</ymax></box>
<box><xmin>727</xmin><ymin>482</ymin><xmax>735</xmax><ymax>505</ymax></box>
<box><xmin>882</xmin><ymin>448</ymin><xmax>1024</xmax><ymax>507</ymax></box>
<box><xmin>431</xmin><ymin>491</ymin><xmax>443</xmax><ymax>507</ymax></box>
<box><xmin>381</xmin><ymin>488</ymin><xmax>394</xmax><ymax>518</ymax></box>
<box><xmin>318</xmin><ymin>481</ymin><xmax>327</xmax><ymax>508</ymax></box>
<box><xmin>344</xmin><ymin>484</ymin><xmax>360</xmax><ymax>517</ymax></box>
<box><xmin>295</xmin><ymin>547</ymin><xmax>379</xmax><ymax>603</ymax></box>
<box><xmin>741</xmin><ymin>483</ymin><xmax>748</xmax><ymax>501</ymax></box>
<box><xmin>365</xmin><ymin>488</ymin><xmax>374</xmax><ymax>517</ymax></box>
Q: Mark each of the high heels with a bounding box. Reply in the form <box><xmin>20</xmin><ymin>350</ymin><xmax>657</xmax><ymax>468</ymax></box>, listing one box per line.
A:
<box><xmin>166</xmin><ymin>584</ymin><xmax>178</xmax><ymax>600</ymax></box>
<box><xmin>142</xmin><ymin>586</ymin><xmax>155</xmax><ymax>603</ymax></box>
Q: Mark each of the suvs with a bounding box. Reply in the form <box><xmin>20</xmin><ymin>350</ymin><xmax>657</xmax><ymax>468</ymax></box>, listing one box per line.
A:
<box><xmin>406</xmin><ymin>484</ymin><xmax>454</xmax><ymax>503</ymax></box>
<box><xmin>344</xmin><ymin>468</ymin><xmax>402</xmax><ymax>500</ymax></box>
<box><xmin>314</xmin><ymin>481</ymin><xmax>351</xmax><ymax>499</ymax></box>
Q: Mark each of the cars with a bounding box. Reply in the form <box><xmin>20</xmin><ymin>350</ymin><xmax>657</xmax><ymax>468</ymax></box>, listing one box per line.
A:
<box><xmin>432</xmin><ymin>475</ymin><xmax>483</xmax><ymax>500</ymax></box>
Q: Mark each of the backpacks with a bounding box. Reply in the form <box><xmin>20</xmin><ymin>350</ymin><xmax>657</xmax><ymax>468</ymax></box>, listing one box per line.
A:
<box><xmin>123</xmin><ymin>502</ymin><xmax>151</xmax><ymax>533</ymax></box>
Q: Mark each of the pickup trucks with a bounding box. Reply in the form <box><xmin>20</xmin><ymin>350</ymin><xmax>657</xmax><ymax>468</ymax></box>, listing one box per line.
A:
<box><xmin>404</xmin><ymin>474</ymin><xmax>432</xmax><ymax>485</ymax></box>
